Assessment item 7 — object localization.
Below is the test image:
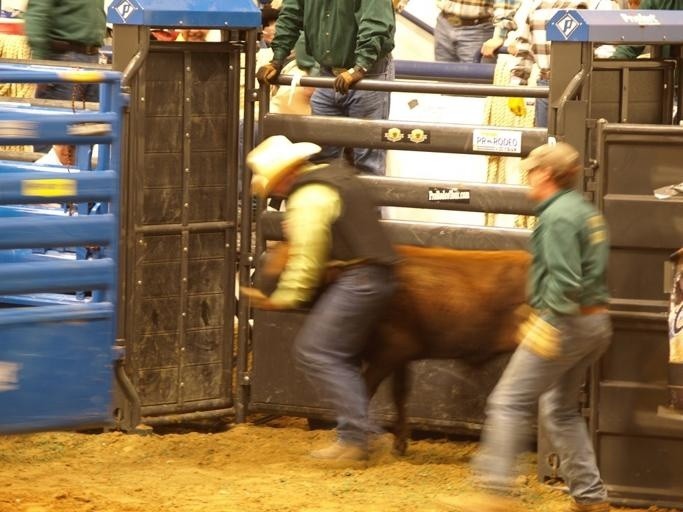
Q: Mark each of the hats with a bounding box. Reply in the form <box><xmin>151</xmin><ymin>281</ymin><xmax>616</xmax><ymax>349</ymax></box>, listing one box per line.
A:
<box><xmin>521</xmin><ymin>143</ymin><xmax>579</xmax><ymax>175</ymax></box>
<box><xmin>246</xmin><ymin>134</ymin><xmax>322</xmax><ymax>198</ymax></box>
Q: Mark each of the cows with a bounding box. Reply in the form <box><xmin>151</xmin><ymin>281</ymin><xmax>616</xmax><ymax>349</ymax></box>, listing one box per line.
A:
<box><xmin>256</xmin><ymin>241</ymin><xmax>540</xmax><ymax>454</ymax></box>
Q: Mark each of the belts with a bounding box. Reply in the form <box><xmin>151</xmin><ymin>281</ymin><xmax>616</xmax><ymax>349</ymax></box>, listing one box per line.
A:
<box><xmin>441</xmin><ymin>13</ymin><xmax>490</xmax><ymax>27</ymax></box>
<box><xmin>48</xmin><ymin>41</ymin><xmax>104</xmax><ymax>54</ymax></box>
<box><xmin>581</xmin><ymin>304</ymin><xmax>607</xmax><ymax>312</ymax></box>
<box><xmin>334</xmin><ymin>66</ymin><xmax>366</xmax><ymax>95</ymax></box>
<box><xmin>328</xmin><ymin>66</ymin><xmax>349</xmax><ymax>77</ymax></box>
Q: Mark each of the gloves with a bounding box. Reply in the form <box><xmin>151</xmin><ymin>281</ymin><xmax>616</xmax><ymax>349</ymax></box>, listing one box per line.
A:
<box><xmin>257</xmin><ymin>63</ymin><xmax>281</xmax><ymax>87</ymax></box>
<box><xmin>516</xmin><ymin>316</ymin><xmax>561</xmax><ymax>358</ymax></box>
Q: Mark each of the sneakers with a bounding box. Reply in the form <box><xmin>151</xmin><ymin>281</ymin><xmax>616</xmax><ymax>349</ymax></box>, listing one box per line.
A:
<box><xmin>569</xmin><ymin>503</ymin><xmax>612</xmax><ymax>511</ymax></box>
<box><xmin>313</xmin><ymin>442</ymin><xmax>368</xmax><ymax>469</ymax></box>
<box><xmin>439</xmin><ymin>491</ymin><xmax>512</xmax><ymax>512</ymax></box>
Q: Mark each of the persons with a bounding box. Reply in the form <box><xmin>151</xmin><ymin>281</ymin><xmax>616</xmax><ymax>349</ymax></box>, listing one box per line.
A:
<box><xmin>23</xmin><ymin>1</ymin><xmax>113</xmax><ymax>259</ymax></box>
<box><xmin>240</xmin><ymin>135</ymin><xmax>401</xmax><ymax>466</ymax></box>
<box><xmin>466</xmin><ymin>141</ymin><xmax>614</xmax><ymax>512</ymax></box>
<box><xmin>154</xmin><ymin>2</ymin><xmax>407</xmax><ymax>220</ymax></box>
<box><xmin>434</xmin><ymin>0</ymin><xmax>683</xmax><ymax>230</ymax></box>
